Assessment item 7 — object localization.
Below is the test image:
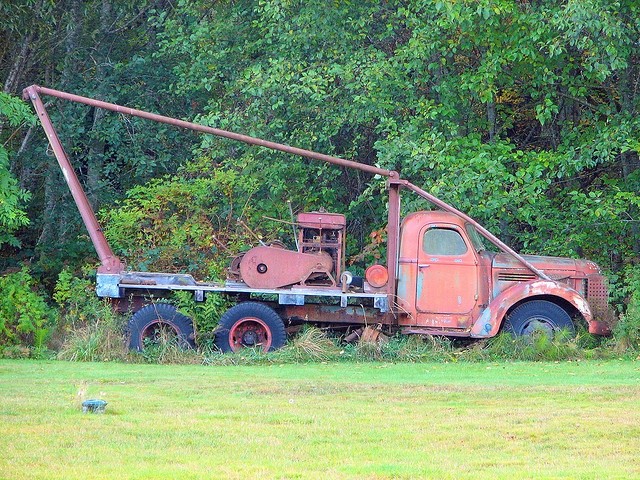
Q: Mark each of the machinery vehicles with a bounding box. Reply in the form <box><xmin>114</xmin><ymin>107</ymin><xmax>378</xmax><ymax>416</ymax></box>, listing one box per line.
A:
<box><xmin>23</xmin><ymin>85</ymin><xmax>615</xmax><ymax>355</ymax></box>
